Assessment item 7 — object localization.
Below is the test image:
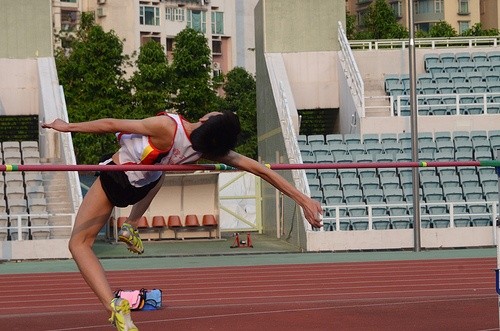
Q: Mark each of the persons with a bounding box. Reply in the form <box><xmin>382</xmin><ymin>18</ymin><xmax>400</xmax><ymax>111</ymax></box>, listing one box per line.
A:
<box><xmin>41</xmin><ymin>111</ymin><xmax>324</xmax><ymax>331</ymax></box>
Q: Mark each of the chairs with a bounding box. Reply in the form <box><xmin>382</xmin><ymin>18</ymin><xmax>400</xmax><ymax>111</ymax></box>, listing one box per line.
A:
<box><xmin>0</xmin><ymin>141</ymin><xmax>50</xmax><ymax>240</ymax></box>
<box><xmin>296</xmin><ymin>130</ymin><xmax>500</xmax><ymax>232</ymax></box>
<box><xmin>385</xmin><ymin>52</ymin><xmax>500</xmax><ymax>116</ymax></box>
<box><xmin>110</xmin><ymin>215</ymin><xmax>218</xmax><ymax>242</ymax></box>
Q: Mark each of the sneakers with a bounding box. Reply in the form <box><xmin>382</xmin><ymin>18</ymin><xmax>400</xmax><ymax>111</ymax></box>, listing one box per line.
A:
<box><xmin>118</xmin><ymin>222</ymin><xmax>144</xmax><ymax>255</ymax></box>
<box><xmin>108</xmin><ymin>298</ymin><xmax>139</xmax><ymax>331</ymax></box>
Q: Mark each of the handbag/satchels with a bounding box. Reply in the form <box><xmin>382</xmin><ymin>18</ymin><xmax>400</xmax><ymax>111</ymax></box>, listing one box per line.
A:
<box><xmin>141</xmin><ymin>288</ymin><xmax>162</xmax><ymax>310</ymax></box>
<box><xmin>114</xmin><ymin>289</ymin><xmax>142</xmax><ymax>309</ymax></box>
